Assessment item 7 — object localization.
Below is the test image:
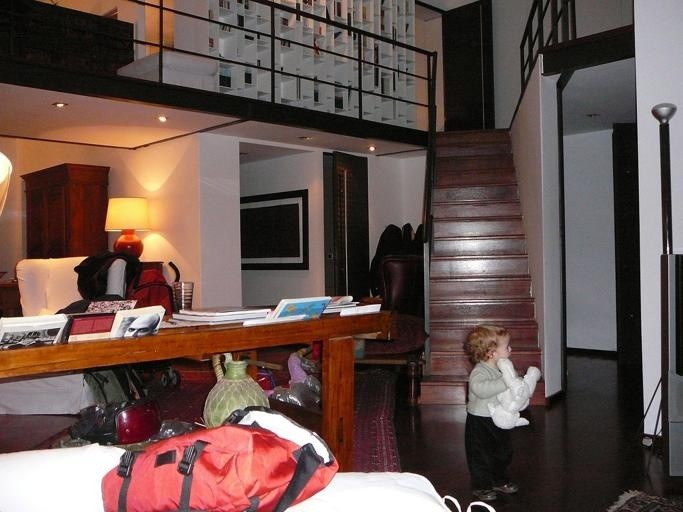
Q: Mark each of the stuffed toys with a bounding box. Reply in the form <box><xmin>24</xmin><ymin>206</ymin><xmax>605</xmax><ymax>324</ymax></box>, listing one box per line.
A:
<box><xmin>486</xmin><ymin>358</ymin><xmax>542</xmax><ymax>432</ymax></box>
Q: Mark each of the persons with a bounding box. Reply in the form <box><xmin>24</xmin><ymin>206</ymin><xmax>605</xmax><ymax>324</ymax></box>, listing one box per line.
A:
<box><xmin>124</xmin><ymin>313</ymin><xmax>159</xmax><ymax>339</ymax></box>
<box><xmin>461</xmin><ymin>323</ymin><xmax>520</xmax><ymax>501</ymax></box>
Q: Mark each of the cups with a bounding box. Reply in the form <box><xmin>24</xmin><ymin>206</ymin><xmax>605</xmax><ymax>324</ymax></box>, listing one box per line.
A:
<box><xmin>171</xmin><ymin>280</ymin><xmax>195</xmax><ymax>314</ymax></box>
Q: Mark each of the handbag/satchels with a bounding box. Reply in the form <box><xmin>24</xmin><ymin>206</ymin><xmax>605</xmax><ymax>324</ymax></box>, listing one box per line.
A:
<box><xmin>82</xmin><ymin>366</ymin><xmax>146</xmax><ymax>409</ymax></box>
<box><xmin>99</xmin><ymin>404</ymin><xmax>340</xmax><ymax>512</ymax></box>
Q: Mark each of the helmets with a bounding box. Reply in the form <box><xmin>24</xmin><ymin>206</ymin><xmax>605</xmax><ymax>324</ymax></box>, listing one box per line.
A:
<box><xmin>69</xmin><ymin>397</ymin><xmax>161</xmax><ymax>446</ymax></box>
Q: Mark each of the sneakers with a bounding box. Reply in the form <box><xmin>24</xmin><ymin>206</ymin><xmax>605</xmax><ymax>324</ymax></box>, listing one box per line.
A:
<box><xmin>492</xmin><ymin>479</ymin><xmax>519</xmax><ymax>494</ymax></box>
<box><xmin>471</xmin><ymin>487</ymin><xmax>498</xmax><ymax>502</ymax></box>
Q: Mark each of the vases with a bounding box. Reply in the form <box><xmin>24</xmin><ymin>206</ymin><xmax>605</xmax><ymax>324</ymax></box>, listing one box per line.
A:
<box><xmin>202</xmin><ymin>359</ymin><xmax>269</xmax><ymax>430</ymax></box>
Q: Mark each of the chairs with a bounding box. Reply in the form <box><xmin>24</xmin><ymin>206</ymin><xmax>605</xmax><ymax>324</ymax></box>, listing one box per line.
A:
<box><xmin>15</xmin><ymin>254</ymin><xmax>90</xmax><ymax>317</ymax></box>
<box><xmin>356</xmin><ymin>222</ymin><xmax>429</xmax><ymax>413</ymax></box>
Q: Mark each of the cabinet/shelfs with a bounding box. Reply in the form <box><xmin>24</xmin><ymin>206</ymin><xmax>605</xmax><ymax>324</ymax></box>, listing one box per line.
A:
<box><xmin>19</xmin><ymin>160</ymin><xmax>114</xmax><ymax>262</ymax></box>
<box><xmin>172</xmin><ymin>0</ymin><xmax>416</xmax><ymax>134</ymax></box>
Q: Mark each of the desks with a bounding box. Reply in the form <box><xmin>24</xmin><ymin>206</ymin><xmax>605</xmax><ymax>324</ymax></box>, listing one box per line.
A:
<box><xmin>1</xmin><ymin>305</ymin><xmax>401</xmax><ymax>476</ymax></box>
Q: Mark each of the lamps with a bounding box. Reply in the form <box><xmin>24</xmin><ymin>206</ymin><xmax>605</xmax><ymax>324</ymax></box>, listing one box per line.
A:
<box><xmin>104</xmin><ymin>196</ymin><xmax>150</xmax><ymax>261</ymax></box>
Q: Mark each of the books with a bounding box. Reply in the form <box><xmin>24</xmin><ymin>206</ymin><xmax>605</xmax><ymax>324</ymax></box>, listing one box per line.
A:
<box><xmin>270</xmin><ymin>295</ymin><xmax>332</xmax><ymax>320</ymax></box>
<box><xmin>168</xmin><ymin>307</ymin><xmax>271</xmax><ymax>327</ymax></box>
<box><xmin>108</xmin><ymin>305</ymin><xmax>167</xmax><ymax>339</ymax></box>
<box><xmin>60</xmin><ymin>313</ymin><xmax>117</xmax><ymax>343</ymax></box>
<box><xmin>324</xmin><ymin>294</ymin><xmax>382</xmax><ymax>318</ymax></box>
<box><xmin>0</xmin><ymin>312</ymin><xmax>66</xmax><ymax>351</ymax></box>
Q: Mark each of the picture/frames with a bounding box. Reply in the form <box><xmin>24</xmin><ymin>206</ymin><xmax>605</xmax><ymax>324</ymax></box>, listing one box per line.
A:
<box><xmin>236</xmin><ymin>187</ymin><xmax>311</xmax><ymax>273</ymax></box>
